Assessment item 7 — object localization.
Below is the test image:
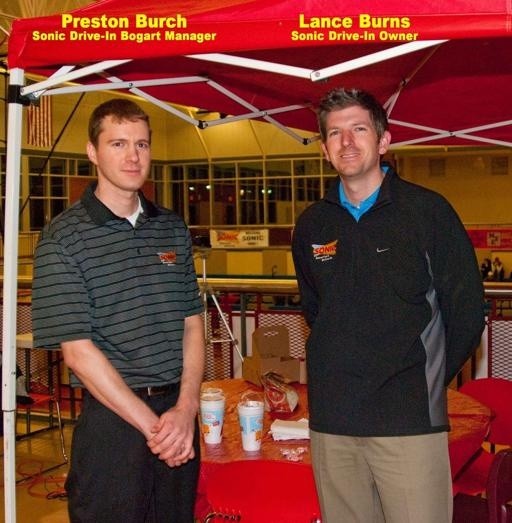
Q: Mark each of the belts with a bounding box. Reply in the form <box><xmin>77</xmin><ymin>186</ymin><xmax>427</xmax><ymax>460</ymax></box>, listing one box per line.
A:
<box><xmin>80</xmin><ymin>382</ymin><xmax>181</xmax><ymax>399</ymax></box>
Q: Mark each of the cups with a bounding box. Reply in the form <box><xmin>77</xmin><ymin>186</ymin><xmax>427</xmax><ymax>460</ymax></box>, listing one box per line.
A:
<box><xmin>199</xmin><ymin>388</ymin><xmax>226</xmax><ymax>444</ymax></box>
<box><xmin>237</xmin><ymin>401</ymin><xmax>265</xmax><ymax>452</ymax></box>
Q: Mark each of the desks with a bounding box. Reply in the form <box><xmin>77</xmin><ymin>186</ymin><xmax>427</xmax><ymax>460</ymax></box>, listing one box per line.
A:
<box><xmin>196</xmin><ymin>379</ymin><xmax>494</xmax><ymax>523</ymax></box>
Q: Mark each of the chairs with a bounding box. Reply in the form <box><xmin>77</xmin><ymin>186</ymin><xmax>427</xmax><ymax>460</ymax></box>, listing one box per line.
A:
<box><xmin>453</xmin><ymin>378</ymin><xmax>511</xmax><ymax>523</ymax></box>
<box><xmin>17</xmin><ymin>365</ymin><xmax>70</xmax><ymax>485</ymax></box>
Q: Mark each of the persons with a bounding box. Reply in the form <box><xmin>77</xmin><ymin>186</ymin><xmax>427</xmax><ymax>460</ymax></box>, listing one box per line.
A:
<box><xmin>289</xmin><ymin>86</ymin><xmax>485</xmax><ymax>523</ymax></box>
<box><xmin>479</xmin><ymin>255</ymin><xmax>505</xmax><ymax>281</ymax></box>
<box><xmin>30</xmin><ymin>98</ymin><xmax>208</xmax><ymax>523</ymax></box>
